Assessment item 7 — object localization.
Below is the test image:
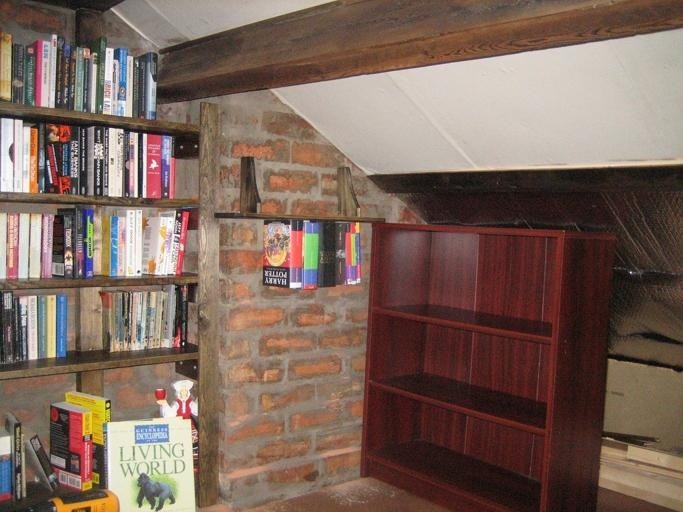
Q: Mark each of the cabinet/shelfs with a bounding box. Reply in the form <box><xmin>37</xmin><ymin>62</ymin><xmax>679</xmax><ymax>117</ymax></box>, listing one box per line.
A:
<box><xmin>360</xmin><ymin>218</ymin><xmax>616</xmax><ymax>512</ymax></box>
<box><xmin>0</xmin><ymin>100</ymin><xmax>220</xmax><ymax>512</ymax></box>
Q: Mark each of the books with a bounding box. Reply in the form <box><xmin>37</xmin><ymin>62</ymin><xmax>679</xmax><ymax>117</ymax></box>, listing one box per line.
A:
<box><xmin>1</xmin><ymin>412</ymin><xmax>27</xmax><ymax>508</ymax></box>
<box><xmin>47</xmin><ymin>400</ymin><xmax>93</xmax><ymax>492</ymax></box>
<box><xmin>0</xmin><ymin>207</ymin><xmax>190</xmax><ymax>280</ymax></box>
<box><xmin>64</xmin><ymin>390</ymin><xmax>111</xmax><ymax>494</ymax></box>
<box><xmin>25</xmin><ymin>434</ymin><xmax>67</xmax><ymax>506</ymax></box>
<box><xmin>0</xmin><ymin>116</ymin><xmax>175</xmax><ymax>199</ymax></box>
<box><xmin>0</xmin><ymin>33</ymin><xmax>160</xmax><ymax>120</ymax></box>
<box><xmin>0</xmin><ymin>284</ymin><xmax>189</xmax><ymax>368</ymax></box>
<box><xmin>102</xmin><ymin>417</ymin><xmax>196</xmax><ymax>512</ymax></box>
<box><xmin>263</xmin><ymin>219</ymin><xmax>362</xmax><ymax>290</ymax></box>
